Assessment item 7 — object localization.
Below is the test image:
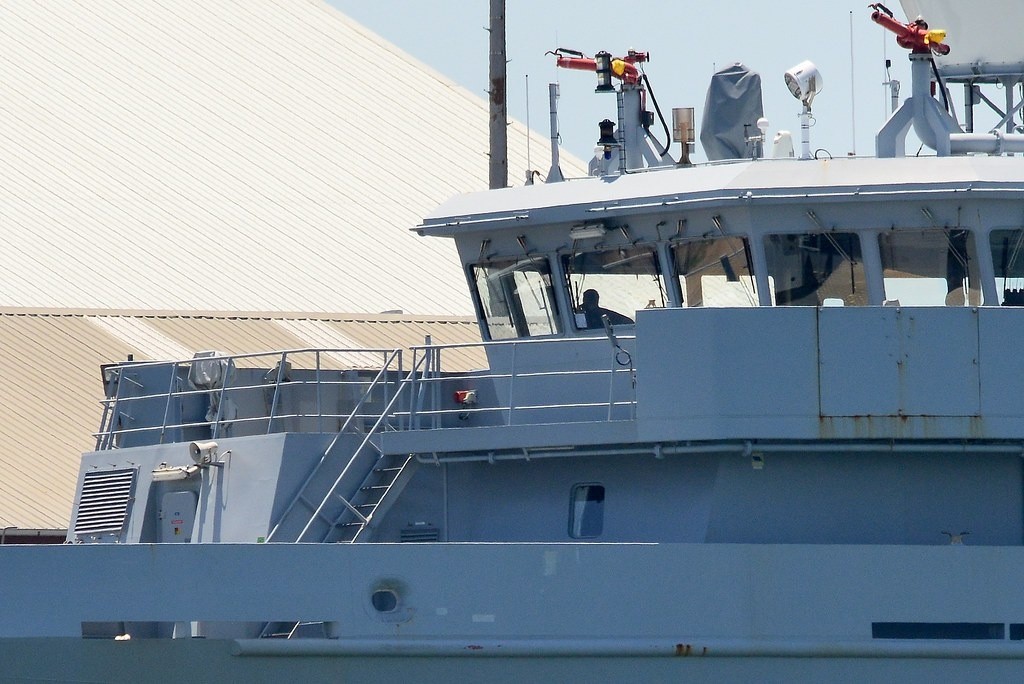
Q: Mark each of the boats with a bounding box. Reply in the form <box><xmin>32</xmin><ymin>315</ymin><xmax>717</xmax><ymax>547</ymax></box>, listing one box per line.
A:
<box><xmin>0</xmin><ymin>0</ymin><xmax>1024</xmax><ymax>682</ymax></box>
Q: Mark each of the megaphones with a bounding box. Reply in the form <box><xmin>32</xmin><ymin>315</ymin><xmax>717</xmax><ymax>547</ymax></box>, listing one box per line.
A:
<box><xmin>189</xmin><ymin>442</ymin><xmax>220</xmax><ymax>464</ymax></box>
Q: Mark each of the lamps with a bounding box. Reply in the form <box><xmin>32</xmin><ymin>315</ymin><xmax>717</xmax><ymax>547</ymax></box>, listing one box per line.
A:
<box><xmin>569</xmin><ymin>221</ymin><xmax>607</xmax><ymax>241</ymax></box>
<box><xmin>784</xmin><ymin>60</ymin><xmax>824</xmax><ymax>157</ymax></box>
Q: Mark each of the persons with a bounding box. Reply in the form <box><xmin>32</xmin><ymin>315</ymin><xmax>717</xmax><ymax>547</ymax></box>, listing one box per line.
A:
<box><xmin>577</xmin><ymin>288</ymin><xmax>634</xmax><ymax>330</ymax></box>
